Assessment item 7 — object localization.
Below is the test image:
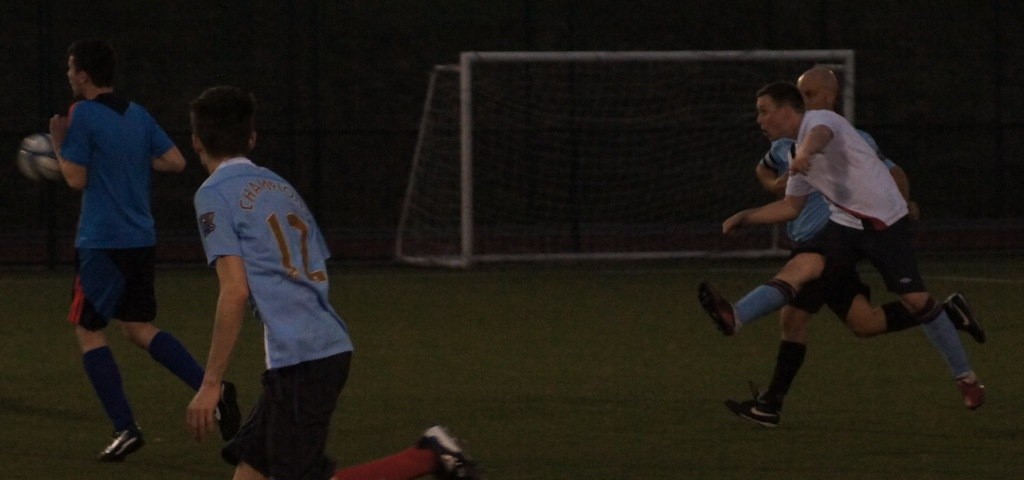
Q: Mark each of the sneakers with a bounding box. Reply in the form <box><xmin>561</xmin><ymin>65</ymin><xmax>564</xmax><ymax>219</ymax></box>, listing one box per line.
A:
<box><xmin>698</xmin><ymin>280</ymin><xmax>743</xmax><ymax>337</ymax></box>
<box><xmin>725</xmin><ymin>393</ymin><xmax>780</xmax><ymax>428</ymax></box>
<box><xmin>96</xmin><ymin>421</ymin><xmax>145</xmax><ymax>463</ymax></box>
<box><xmin>944</xmin><ymin>292</ymin><xmax>988</xmax><ymax>344</ymax></box>
<box><xmin>414</xmin><ymin>424</ymin><xmax>486</xmax><ymax>479</ymax></box>
<box><xmin>211</xmin><ymin>380</ymin><xmax>241</xmax><ymax>441</ymax></box>
<box><xmin>955</xmin><ymin>369</ymin><xmax>985</xmax><ymax>410</ymax></box>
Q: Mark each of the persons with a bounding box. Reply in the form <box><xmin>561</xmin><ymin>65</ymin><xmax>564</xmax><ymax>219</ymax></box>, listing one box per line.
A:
<box><xmin>50</xmin><ymin>43</ymin><xmax>242</xmax><ymax>461</ymax></box>
<box><xmin>724</xmin><ymin>68</ymin><xmax>986</xmax><ymax>427</ymax></box>
<box><xmin>185</xmin><ymin>86</ymin><xmax>481</xmax><ymax>480</ymax></box>
<box><xmin>696</xmin><ymin>80</ymin><xmax>985</xmax><ymax>409</ymax></box>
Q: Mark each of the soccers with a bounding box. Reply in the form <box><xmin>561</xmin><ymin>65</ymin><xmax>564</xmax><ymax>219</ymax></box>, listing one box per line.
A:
<box><xmin>18</xmin><ymin>132</ymin><xmax>61</xmax><ymax>183</ymax></box>
